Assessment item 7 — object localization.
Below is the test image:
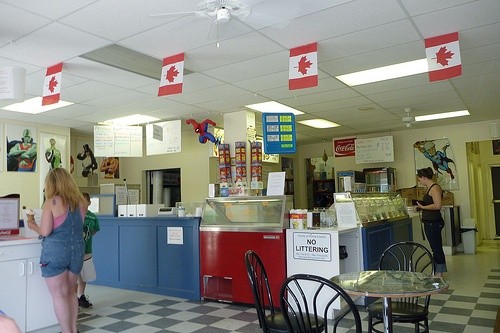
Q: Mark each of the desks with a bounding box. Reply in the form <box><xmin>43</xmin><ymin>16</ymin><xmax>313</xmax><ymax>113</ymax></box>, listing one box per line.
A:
<box><xmin>329</xmin><ymin>270</ymin><xmax>448</xmax><ymax>333</ymax></box>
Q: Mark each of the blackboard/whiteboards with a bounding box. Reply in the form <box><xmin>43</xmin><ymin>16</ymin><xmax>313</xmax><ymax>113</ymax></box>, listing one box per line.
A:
<box><xmin>354</xmin><ymin>135</ymin><xmax>394</xmax><ymax>164</ymax></box>
<box><xmin>94</xmin><ymin>125</ymin><xmax>143</xmax><ymax>157</ymax></box>
<box><xmin>145</xmin><ymin>119</ymin><xmax>182</xmax><ymax>156</ymax></box>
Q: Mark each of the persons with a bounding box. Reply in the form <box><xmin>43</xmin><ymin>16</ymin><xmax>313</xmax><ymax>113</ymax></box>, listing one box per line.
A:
<box><xmin>415</xmin><ymin>166</ymin><xmax>448</xmax><ymax>280</ymax></box>
<box><xmin>26</xmin><ymin>167</ymin><xmax>86</xmax><ymax>333</ymax></box>
<box><xmin>74</xmin><ymin>192</ymin><xmax>101</xmax><ymax>309</ymax></box>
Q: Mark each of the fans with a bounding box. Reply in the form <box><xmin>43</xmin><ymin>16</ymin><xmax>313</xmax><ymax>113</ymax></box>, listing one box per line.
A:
<box><xmin>149</xmin><ymin>0</ymin><xmax>293</xmax><ymax>47</ymax></box>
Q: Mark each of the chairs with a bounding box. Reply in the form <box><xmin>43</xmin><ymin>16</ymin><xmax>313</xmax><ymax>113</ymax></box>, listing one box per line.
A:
<box><xmin>244</xmin><ymin>241</ymin><xmax>435</xmax><ymax>333</ymax></box>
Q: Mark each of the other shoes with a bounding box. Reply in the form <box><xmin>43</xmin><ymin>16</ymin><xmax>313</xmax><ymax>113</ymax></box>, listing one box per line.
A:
<box><xmin>78</xmin><ymin>294</ymin><xmax>93</xmax><ymax>309</ymax></box>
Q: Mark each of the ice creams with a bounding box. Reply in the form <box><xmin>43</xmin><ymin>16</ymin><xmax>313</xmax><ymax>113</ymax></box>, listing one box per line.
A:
<box><xmin>26</xmin><ymin>209</ymin><xmax>34</xmax><ymax>222</ymax></box>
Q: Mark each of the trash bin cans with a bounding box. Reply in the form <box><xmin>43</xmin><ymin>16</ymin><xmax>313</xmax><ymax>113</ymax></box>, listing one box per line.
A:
<box><xmin>460</xmin><ymin>218</ymin><xmax>478</xmax><ymax>254</ymax></box>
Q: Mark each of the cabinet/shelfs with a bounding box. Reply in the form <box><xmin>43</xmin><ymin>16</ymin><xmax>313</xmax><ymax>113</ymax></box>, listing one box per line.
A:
<box><xmin>86</xmin><ymin>217</ymin><xmax>202</xmax><ymax>302</ymax></box>
<box><xmin>361</xmin><ymin>218</ymin><xmax>413</xmax><ymax>305</ymax></box>
<box><xmin>0</xmin><ymin>240</ymin><xmax>59</xmax><ymax>333</ymax></box>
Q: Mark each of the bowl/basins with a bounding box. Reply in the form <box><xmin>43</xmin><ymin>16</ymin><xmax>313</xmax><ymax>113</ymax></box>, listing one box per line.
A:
<box><xmin>177</xmin><ymin>207</ymin><xmax>186</xmax><ymax>218</ymax></box>
